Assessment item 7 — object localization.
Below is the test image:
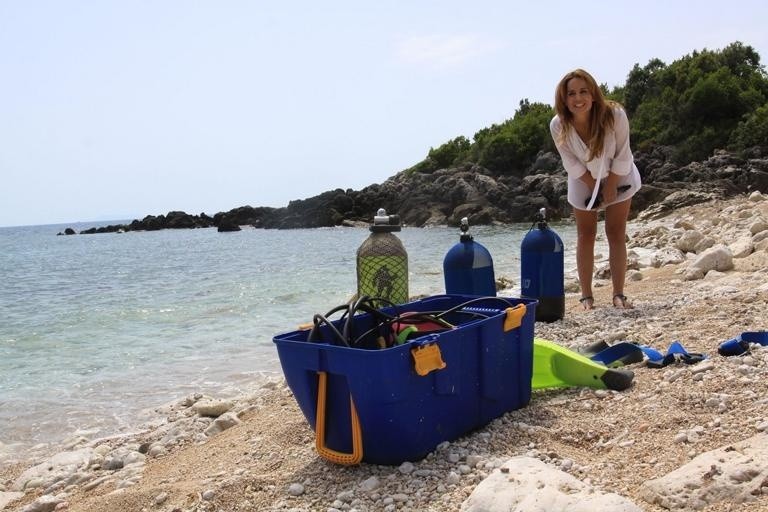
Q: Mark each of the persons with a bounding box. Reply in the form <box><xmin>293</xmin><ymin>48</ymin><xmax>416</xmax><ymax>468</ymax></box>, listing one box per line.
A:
<box><xmin>549</xmin><ymin>70</ymin><xmax>641</xmax><ymax>310</ymax></box>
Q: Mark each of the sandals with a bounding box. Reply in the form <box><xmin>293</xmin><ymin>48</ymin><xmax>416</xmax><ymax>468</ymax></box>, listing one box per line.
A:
<box><xmin>579</xmin><ymin>296</ymin><xmax>596</xmax><ymax>311</ymax></box>
<box><xmin>611</xmin><ymin>293</ymin><xmax>635</xmax><ymax>311</ymax></box>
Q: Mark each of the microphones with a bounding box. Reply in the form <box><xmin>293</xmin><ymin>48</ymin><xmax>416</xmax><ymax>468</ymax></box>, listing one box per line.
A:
<box><xmin>585</xmin><ymin>186</ymin><xmax>631</xmax><ymax>208</ymax></box>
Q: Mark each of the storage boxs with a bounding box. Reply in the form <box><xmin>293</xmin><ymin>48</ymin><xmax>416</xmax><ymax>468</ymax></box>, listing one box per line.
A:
<box><xmin>272</xmin><ymin>294</ymin><xmax>541</xmax><ymax>466</ymax></box>
